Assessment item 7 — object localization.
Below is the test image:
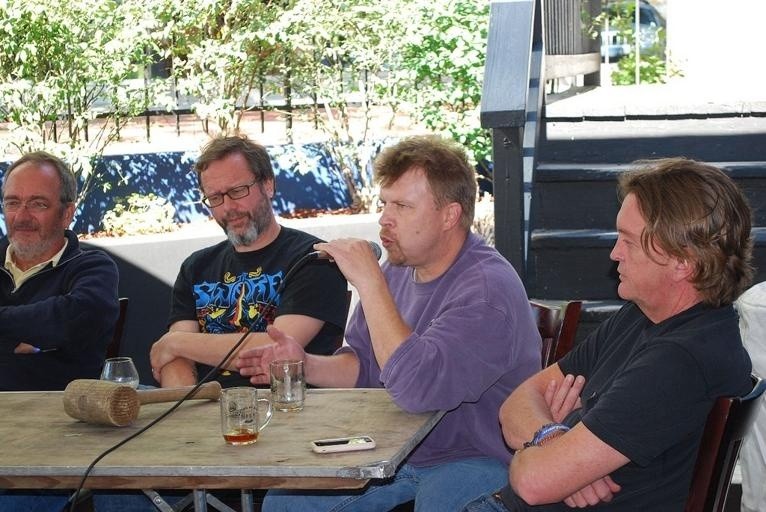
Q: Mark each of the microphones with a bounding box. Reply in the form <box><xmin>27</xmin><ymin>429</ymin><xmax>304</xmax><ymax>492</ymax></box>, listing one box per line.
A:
<box><xmin>316</xmin><ymin>241</ymin><xmax>383</xmax><ymax>263</ymax></box>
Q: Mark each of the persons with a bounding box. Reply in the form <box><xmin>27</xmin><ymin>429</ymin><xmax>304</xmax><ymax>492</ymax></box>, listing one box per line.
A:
<box><xmin>464</xmin><ymin>156</ymin><xmax>758</xmax><ymax>512</ymax></box>
<box><xmin>88</xmin><ymin>132</ymin><xmax>350</xmax><ymax>510</ymax></box>
<box><xmin>233</xmin><ymin>134</ymin><xmax>546</xmax><ymax>511</ymax></box>
<box><xmin>0</xmin><ymin>149</ymin><xmax>121</xmax><ymax>511</ymax></box>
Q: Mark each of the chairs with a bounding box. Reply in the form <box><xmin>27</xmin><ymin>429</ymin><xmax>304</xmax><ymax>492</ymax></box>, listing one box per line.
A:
<box><xmin>686</xmin><ymin>372</ymin><xmax>766</xmax><ymax>512</ymax></box>
<box><xmin>97</xmin><ymin>297</ymin><xmax>129</xmax><ymax>382</ymax></box>
<box><xmin>526</xmin><ymin>299</ymin><xmax>582</xmax><ymax>369</ymax></box>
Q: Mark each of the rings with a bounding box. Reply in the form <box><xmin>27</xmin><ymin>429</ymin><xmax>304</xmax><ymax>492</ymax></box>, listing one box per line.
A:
<box><xmin>151</xmin><ymin>367</ymin><xmax>156</xmax><ymax>372</ymax></box>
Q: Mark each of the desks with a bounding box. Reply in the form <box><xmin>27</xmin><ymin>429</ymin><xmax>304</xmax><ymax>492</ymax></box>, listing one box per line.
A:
<box><xmin>1</xmin><ymin>387</ymin><xmax>448</xmax><ymax>512</ymax></box>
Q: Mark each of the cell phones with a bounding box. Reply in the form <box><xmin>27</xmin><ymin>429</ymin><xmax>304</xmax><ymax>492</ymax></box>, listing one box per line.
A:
<box><xmin>311</xmin><ymin>436</ymin><xmax>375</xmax><ymax>454</ymax></box>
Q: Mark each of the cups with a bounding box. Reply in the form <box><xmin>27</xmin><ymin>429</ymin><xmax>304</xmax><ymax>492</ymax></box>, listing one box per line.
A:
<box><xmin>100</xmin><ymin>356</ymin><xmax>140</xmax><ymax>390</ymax></box>
<box><xmin>218</xmin><ymin>386</ymin><xmax>273</xmax><ymax>445</ymax></box>
<box><xmin>269</xmin><ymin>359</ymin><xmax>305</xmax><ymax>411</ymax></box>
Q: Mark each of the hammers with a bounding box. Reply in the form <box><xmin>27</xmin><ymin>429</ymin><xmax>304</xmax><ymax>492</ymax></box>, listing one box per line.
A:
<box><xmin>64</xmin><ymin>379</ymin><xmax>222</xmax><ymax>427</ymax></box>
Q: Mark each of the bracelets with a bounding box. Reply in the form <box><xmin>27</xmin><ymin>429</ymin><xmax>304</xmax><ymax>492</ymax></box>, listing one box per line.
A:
<box><xmin>526</xmin><ymin>422</ymin><xmax>570</xmax><ymax>449</ymax></box>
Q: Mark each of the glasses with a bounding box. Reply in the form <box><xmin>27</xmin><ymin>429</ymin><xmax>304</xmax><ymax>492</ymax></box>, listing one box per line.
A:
<box><xmin>202</xmin><ymin>179</ymin><xmax>259</xmax><ymax>209</ymax></box>
<box><xmin>1</xmin><ymin>199</ymin><xmax>62</xmax><ymax>213</ymax></box>
